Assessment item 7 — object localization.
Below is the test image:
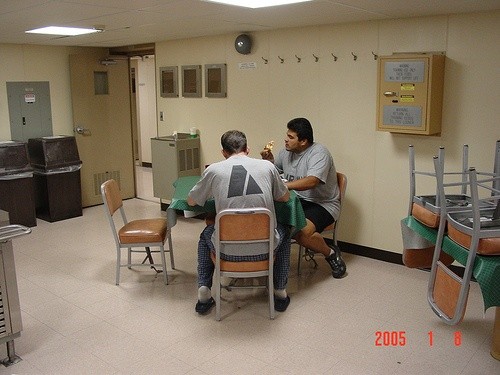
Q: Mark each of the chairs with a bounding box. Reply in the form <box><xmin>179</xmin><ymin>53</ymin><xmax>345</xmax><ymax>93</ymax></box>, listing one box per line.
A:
<box><xmin>210</xmin><ymin>208</ymin><xmax>276</xmax><ymax>321</ymax></box>
<box><xmin>402</xmin><ymin>140</ymin><xmax>500</xmax><ymax>326</ymax></box>
<box><xmin>290</xmin><ymin>173</ymin><xmax>347</xmax><ymax>276</ymax></box>
<box><xmin>101</xmin><ymin>179</ymin><xmax>174</xmax><ymax>286</ymax></box>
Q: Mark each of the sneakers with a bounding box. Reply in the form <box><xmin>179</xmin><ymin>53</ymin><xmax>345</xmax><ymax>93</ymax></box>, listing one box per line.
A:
<box><xmin>325</xmin><ymin>244</ymin><xmax>346</xmax><ymax>277</ymax></box>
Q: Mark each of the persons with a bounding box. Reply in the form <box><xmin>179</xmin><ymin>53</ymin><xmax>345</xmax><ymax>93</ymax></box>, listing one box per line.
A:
<box><xmin>187</xmin><ymin>129</ymin><xmax>292</xmax><ymax>312</ymax></box>
<box><xmin>259</xmin><ymin>117</ymin><xmax>346</xmax><ymax>278</ymax></box>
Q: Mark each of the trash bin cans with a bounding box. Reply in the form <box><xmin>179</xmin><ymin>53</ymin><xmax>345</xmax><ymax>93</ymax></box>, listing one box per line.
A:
<box><xmin>28</xmin><ymin>135</ymin><xmax>85</xmax><ymax>224</ymax></box>
<box><xmin>0</xmin><ymin>138</ymin><xmax>38</xmax><ymax>228</ymax></box>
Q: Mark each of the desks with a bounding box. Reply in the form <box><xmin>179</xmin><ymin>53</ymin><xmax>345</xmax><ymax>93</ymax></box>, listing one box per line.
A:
<box><xmin>400</xmin><ymin>216</ymin><xmax>500</xmax><ymax>362</ymax></box>
<box><xmin>167</xmin><ymin>176</ymin><xmax>307</xmax><ymax>288</ymax></box>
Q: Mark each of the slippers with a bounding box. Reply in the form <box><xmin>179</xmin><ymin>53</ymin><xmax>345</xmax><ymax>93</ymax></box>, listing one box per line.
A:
<box><xmin>195</xmin><ymin>296</ymin><xmax>216</xmax><ymax>312</ymax></box>
<box><xmin>274</xmin><ymin>293</ymin><xmax>290</xmax><ymax>312</ymax></box>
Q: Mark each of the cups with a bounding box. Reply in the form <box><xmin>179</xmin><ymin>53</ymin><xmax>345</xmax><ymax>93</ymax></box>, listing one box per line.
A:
<box><xmin>190</xmin><ymin>128</ymin><xmax>198</xmax><ymax>139</ymax></box>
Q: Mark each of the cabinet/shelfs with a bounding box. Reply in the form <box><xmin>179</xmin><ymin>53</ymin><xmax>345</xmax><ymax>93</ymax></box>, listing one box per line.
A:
<box><xmin>377</xmin><ymin>54</ymin><xmax>446</xmax><ymax>135</ymax></box>
<box><xmin>150</xmin><ymin>137</ymin><xmax>201</xmax><ymax>199</ymax></box>
<box><xmin>0</xmin><ymin>209</ymin><xmax>23</xmax><ymax>368</ymax></box>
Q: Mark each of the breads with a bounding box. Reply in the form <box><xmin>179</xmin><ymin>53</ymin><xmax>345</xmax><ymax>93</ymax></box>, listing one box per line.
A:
<box><xmin>264</xmin><ymin>141</ymin><xmax>274</xmax><ymax>152</ymax></box>
